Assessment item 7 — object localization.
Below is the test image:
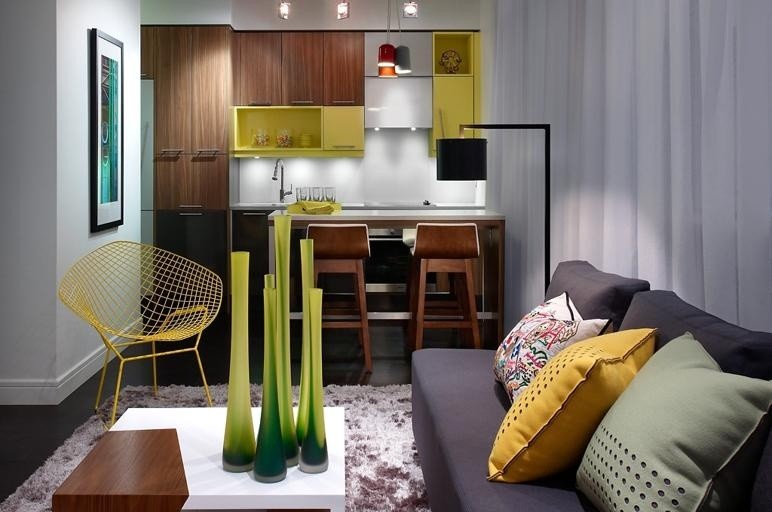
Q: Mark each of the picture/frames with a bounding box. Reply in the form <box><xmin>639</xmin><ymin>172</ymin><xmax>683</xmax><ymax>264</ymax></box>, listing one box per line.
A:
<box><xmin>90</xmin><ymin>26</ymin><xmax>125</xmax><ymax>232</ymax></box>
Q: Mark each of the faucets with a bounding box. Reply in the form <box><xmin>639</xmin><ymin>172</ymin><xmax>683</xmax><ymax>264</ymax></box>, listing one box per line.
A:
<box><xmin>272</xmin><ymin>158</ymin><xmax>284</xmax><ymax>201</ymax></box>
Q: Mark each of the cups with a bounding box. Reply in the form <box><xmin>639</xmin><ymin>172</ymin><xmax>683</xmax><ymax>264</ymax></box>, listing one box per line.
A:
<box><xmin>295</xmin><ymin>187</ymin><xmax>336</xmax><ymax>203</ymax></box>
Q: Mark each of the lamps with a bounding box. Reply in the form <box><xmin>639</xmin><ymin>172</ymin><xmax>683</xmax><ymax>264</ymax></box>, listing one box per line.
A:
<box><xmin>378</xmin><ymin>0</ymin><xmax>412</xmax><ymax>79</ymax></box>
<box><xmin>337</xmin><ymin>0</ymin><xmax>350</xmax><ymax>20</ymax></box>
<box><xmin>277</xmin><ymin>0</ymin><xmax>291</xmax><ymax>20</ymax></box>
<box><xmin>435</xmin><ymin>124</ymin><xmax>551</xmax><ymax>298</ymax></box>
<box><xmin>402</xmin><ymin>0</ymin><xmax>418</xmax><ymax>18</ymax></box>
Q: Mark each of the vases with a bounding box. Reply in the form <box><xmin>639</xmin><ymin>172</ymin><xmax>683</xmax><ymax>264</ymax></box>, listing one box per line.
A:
<box><xmin>222</xmin><ymin>212</ymin><xmax>328</xmax><ymax>486</ymax></box>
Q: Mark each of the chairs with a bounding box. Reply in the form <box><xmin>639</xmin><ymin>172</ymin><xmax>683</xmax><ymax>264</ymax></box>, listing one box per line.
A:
<box><xmin>57</xmin><ymin>240</ymin><xmax>222</xmax><ymax>430</ymax></box>
<box><xmin>409</xmin><ymin>222</ymin><xmax>481</xmax><ymax>350</ymax></box>
<box><xmin>305</xmin><ymin>223</ymin><xmax>373</xmax><ymax>375</ymax></box>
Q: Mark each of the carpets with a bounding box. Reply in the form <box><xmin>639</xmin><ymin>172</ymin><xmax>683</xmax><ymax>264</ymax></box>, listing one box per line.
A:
<box><xmin>1</xmin><ymin>382</ymin><xmax>429</xmax><ymax>512</ymax></box>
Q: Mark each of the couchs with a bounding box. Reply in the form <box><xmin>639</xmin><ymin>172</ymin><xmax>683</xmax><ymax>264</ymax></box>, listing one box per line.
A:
<box><xmin>411</xmin><ymin>262</ymin><xmax>772</xmax><ymax>512</ymax></box>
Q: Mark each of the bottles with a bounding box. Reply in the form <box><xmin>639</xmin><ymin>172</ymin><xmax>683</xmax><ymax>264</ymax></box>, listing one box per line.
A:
<box><xmin>251</xmin><ymin>128</ymin><xmax>271</xmax><ymax>147</ymax></box>
<box><xmin>274</xmin><ymin>127</ymin><xmax>295</xmax><ymax>148</ymax></box>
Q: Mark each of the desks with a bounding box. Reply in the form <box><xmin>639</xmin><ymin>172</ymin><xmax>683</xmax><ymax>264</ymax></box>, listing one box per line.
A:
<box><xmin>267</xmin><ymin>209</ymin><xmax>505</xmax><ymax>346</ymax></box>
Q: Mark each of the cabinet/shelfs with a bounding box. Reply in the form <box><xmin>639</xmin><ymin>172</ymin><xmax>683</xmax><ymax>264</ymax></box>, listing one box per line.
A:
<box><xmin>230</xmin><ymin>210</ymin><xmax>274</xmax><ymax>294</ymax></box>
<box><xmin>229</xmin><ymin>31</ymin><xmax>482</xmax><ymax>159</ymax></box>
<box><xmin>141</xmin><ymin>25</ymin><xmax>231</xmax><ymax>357</ymax></box>
